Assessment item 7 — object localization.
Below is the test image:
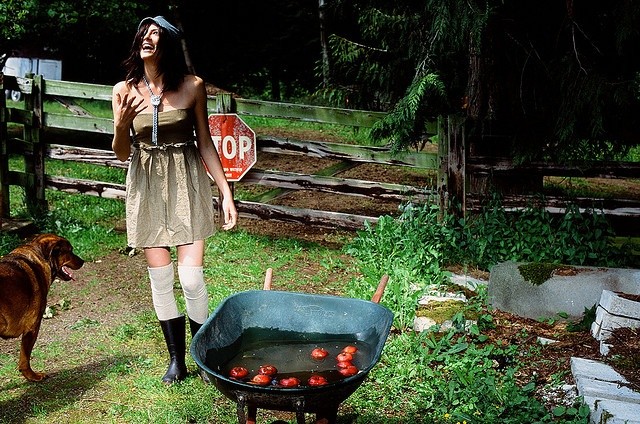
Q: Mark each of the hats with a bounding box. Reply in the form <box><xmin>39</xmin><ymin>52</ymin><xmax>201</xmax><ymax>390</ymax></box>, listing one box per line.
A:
<box><xmin>137</xmin><ymin>15</ymin><xmax>180</xmax><ymax>32</ymax></box>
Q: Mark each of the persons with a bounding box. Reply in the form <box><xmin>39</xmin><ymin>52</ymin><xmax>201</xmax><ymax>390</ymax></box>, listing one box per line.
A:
<box><xmin>111</xmin><ymin>15</ymin><xmax>238</xmax><ymax>384</ymax></box>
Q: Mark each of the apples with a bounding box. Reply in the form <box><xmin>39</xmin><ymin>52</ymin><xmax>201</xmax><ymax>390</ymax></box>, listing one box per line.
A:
<box><xmin>343</xmin><ymin>346</ymin><xmax>358</xmax><ymax>356</ymax></box>
<box><xmin>339</xmin><ymin>365</ymin><xmax>358</xmax><ymax>376</ymax></box>
<box><xmin>258</xmin><ymin>364</ymin><xmax>279</xmax><ymax>377</ymax></box>
<box><xmin>279</xmin><ymin>377</ymin><xmax>300</xmax><ymax>388</ymax></box>
<box><xmin>308</xmin><ymin>374</ymin><xmax>328</xmax><ymax>386</ymax></box>
<box><xmin>253</xmin><ymin>375</ymin><xmax>271</xmax><ymax>386</ymax></box>
<box><xmin>310</xmin><ymin>347</ymin><xmax>328</xmax><ymax>362</ymax></box>
<box><xmin>337</xmin><ymin>361</ymin><xmax>353</xmax><ymax>368</ymax></box>
<box><xmin>338</xmin><ymin>353</ymin><xmax>353</xmax><ymax>363</ymax></box>
<box><xmin>229</xmin><ymin>366</ymin><xmax>249</xmax><ymax>378</ymax></box>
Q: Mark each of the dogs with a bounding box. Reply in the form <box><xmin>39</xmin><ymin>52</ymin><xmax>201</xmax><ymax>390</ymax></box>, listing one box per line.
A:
<box><xmin>0</xmin><ymin>234</ymin><xmax>84</xmax><ymax>382</ymax></box>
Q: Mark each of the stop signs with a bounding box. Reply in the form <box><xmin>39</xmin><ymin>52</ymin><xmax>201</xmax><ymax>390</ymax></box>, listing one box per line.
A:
<box><xmin>195</xmin><ymin>114</ymin><xmax>257</xmax><ymax>181</ymax></box>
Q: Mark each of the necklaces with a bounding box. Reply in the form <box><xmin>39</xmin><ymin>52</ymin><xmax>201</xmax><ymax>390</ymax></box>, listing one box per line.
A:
<box><xmin>142</xmin><ymin>74</ymin><xmax>167</xmax><ymax>146</ymax></box>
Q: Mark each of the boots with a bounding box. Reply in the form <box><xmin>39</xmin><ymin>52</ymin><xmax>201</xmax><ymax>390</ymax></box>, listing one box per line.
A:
<box><xmin>189</xmin><ymin>317</ymin><xmax>203</xmax><ymax>385</ymax></box>
<box><xmin>159</xmin><ymin>314</ymin><xmax>186</xmax><ymax>383</ymax></box>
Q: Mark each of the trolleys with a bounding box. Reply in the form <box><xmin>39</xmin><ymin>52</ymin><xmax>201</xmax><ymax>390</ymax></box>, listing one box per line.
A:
<box><xmin>191</xmin><ymin>268</ymin><xmax>394</xmax><ymax>424</ymax></box>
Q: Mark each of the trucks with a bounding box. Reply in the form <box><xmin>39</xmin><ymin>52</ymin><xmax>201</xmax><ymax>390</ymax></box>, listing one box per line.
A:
<box><xmin>1</xmin><ymin>57</ymin><xmax>62</xmax><ymax>102</ymax></box>
